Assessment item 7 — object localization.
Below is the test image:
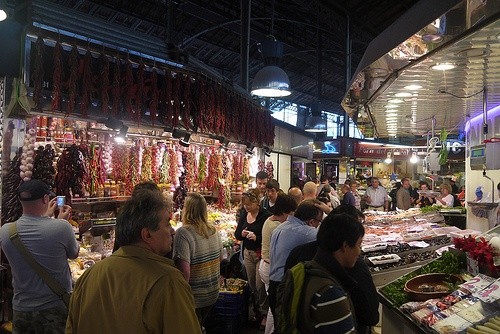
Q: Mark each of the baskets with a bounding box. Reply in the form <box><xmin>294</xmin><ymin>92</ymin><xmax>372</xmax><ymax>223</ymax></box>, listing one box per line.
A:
<box><xmin>404</xmin><ymin>272</ymin><xmax>463</xmax><ymax>301</ymax></box>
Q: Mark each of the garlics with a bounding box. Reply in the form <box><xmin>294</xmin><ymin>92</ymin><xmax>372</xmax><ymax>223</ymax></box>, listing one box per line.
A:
<box><xmin>20</xmin><ymin>129</ymin><xmax>35</xmax><ymax>182</ymax></box>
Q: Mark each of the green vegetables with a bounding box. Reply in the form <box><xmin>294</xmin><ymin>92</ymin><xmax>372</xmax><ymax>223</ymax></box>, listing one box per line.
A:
<box><xmin>380</xmin><ymin>248</ymin><xmax>468</xmax><ymax>306</ymax></box>
<box><xmin>420</xmin><ymin>205</ymin><xmax>466</xmax><ymax>214</ymax></box>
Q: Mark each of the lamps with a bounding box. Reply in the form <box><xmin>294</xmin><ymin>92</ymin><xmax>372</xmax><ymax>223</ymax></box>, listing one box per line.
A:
<box><xmin>263</xmin><ymin>146</ymin><xmax>271</xmax><ymax>157</ymax></box>
<box><xmin>105</xmin><ymin>119</ymin><xmax>129</xmax><ymax>144</ymax></box>
<box><xmin>172</xmin><ymin>128</ymin><xmax>191</xmax><ymax>147</ymax></box>
<box><xmin>246</xmin><ymin>144</ymin><xmax>255</xmax><ymax>155</ymax></box>
<box><xmin>250</xmin><ymin>0</ymin><xmax>293</xmax><ymax>97</ymax></box>
<box><xmin>218</xmin><ymin>139</ymin><xmax>229</xmax><ymax>153</ymax></box>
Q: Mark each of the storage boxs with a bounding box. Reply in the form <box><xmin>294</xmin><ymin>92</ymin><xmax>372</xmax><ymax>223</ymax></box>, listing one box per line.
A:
<box><xmin>213</xmin><ymin>285</ymin><xmax>253</xmax><ymax>334</ymax></box>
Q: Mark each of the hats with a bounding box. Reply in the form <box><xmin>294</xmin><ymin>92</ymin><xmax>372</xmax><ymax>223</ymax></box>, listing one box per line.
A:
<box><xmin>17</xmin><ymin>179</ymin><xmax>56</xmax><ymax>201</ymax></box>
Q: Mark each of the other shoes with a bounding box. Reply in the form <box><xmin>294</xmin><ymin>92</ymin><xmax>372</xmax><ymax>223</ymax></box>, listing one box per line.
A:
<box><xmin>250</xmin><ymin>313</ymin><xmax>266</xmax><ymax>330</ymax></box>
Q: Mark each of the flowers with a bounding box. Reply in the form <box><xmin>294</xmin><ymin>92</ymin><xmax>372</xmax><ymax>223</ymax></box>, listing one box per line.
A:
<box><xmin>453</xmin><ymin>235</ymin><xmax>500</xmax><ymax>277</ymax></box>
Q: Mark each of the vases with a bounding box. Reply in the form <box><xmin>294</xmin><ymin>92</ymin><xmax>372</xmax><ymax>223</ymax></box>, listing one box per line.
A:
<box><xmin>466</xmin><ymin>256</ymin><xmax>479</xmax><ymax>276</ymax></box>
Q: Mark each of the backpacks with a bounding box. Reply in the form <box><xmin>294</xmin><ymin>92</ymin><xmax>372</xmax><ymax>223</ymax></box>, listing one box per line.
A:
<box><xmin>274</xmin><ymin>261</ymin><xmax>335</xmax><ymax>334</ymax></box>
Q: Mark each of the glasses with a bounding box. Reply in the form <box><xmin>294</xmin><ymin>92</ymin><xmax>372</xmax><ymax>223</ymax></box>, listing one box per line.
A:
<box><xmin>310</xmin><ymin>217</ymin><xmax>323</xmax><ymax>224</ymax></box>
<box><xmin>242</xmin><ymin>192</ymin><xmax>256</xmax><ymax>198</ymax></box>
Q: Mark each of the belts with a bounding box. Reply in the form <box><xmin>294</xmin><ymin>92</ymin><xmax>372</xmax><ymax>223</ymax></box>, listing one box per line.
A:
<box><xmin>367</xmin><ymin>205</ymin><xmax>382</xmax><ymax>208</ymax></box>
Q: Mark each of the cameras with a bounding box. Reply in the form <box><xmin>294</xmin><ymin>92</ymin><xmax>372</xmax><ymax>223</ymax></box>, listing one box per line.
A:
<box><xmin>56</xmin><ymin>195</ymin><xmax>66</xmax><ymax>209</ymax></box>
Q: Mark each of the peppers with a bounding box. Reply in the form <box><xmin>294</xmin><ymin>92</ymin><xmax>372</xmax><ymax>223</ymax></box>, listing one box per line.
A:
<box><xmin>79</xmin><ymin>142</ymin><xmax>106</xmax><ymax>196</ymax></box>
<box><xmin>111</xmin><ymin>145</ymin><xmax>250</xmax><ymax>212</ymax></box>
<box><xmin>33</xmin><ymin>35</ymin><xmax>275</xmax><ymax>147</ymax></box>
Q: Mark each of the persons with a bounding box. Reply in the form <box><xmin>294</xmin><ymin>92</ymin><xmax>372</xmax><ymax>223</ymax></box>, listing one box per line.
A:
<box><xmin>284</xmin><ymin>203</ymin><xmax>380</xmax><ymax>334</ymax></box>
<box><xmin>396</xmin><ymin>180</ymin><xmax>410</xmax><ymax>210</ymax></box>
<box><xmin>258</xmin><ymin>194</ymin><xmax>298</xmax><ymax>334</ymax></box>
<box><xmin>234</xmin><ymin>189</ymin><xmax>269</xmax><ymax>331</ymax></box>
<box><xmin>267</xmin><ymin>198</ymin><xmax>333</xmax><ymax>334</ymax></box>
<box><xmin>0</xmin><ymin>180</ymin><xmax>80</xmax><ymax>334</ymax></box>
<box><xmin>296</xmin><ymin>213</ymin><xmax>365</xmax><ymax>334</ymax></box>
<box><xmin>388</xmin><ymin>182</ymin><xmax>403</xmax><ymax>210</ymax></box>
<box><xmin>408</xmin><ymin>178</ymin><xmax>465</xmax><ymax>208</ymax></box>
<box><xmin>172</xmin><ymin>192</ymin><xmax>224</xmax><ymax>334</ymax></box>
<box><xmin>365</xmin><ymin>177</ymin><xmax>382</xmax><ymax>209</ymax></box>
<box><xmin>65</xmin><ymin>190</ymin><xmax>203</xmax><ymax>334</ymax></box>
<box><xmin>112</xmin><ymin>182</ymin><xmax>174</xmax><ymax>260</ymax></box>
<box><xmin>235</xmin><ymin>171</ymin><xmax>361</xmax><ymax>224</ymax></box>
<box><xmin>362</xmin><ymin>178</ymin><xmax>388</xmax><ymax>212</ymax></box>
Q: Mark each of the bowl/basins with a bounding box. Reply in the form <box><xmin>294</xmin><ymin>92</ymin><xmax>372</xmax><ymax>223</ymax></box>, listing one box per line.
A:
<box><xmin>404</xmin><ymin>274</ymin><xmax>461</xmax><ymax>300</ymax></box>
